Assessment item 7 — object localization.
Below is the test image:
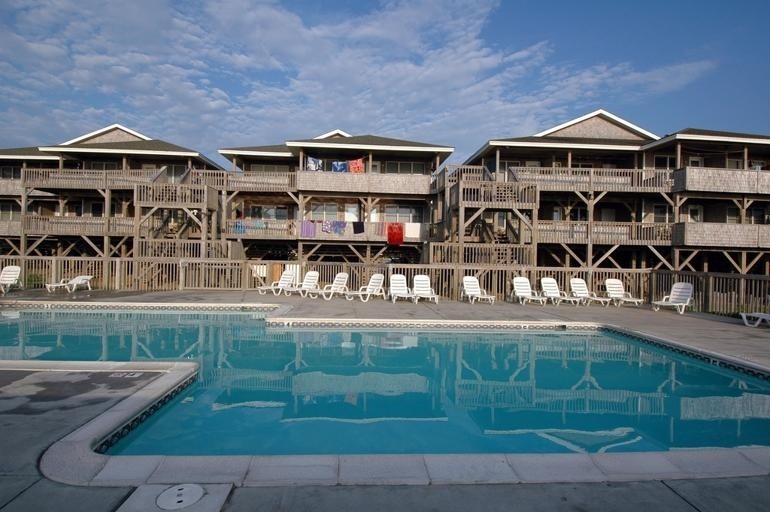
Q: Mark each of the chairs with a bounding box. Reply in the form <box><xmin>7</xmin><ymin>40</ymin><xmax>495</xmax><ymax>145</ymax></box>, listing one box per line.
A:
<box><xmin>651</xmin><ymin>282</ymin><xmax>696</xmax><ymax>315</ymax></box>
<box><xmin>459</xmin><ymin>275</ymin><xmax>495</xmax><ymax>305</ymax></box>
<box><xmin>510</xmin><ymin>276</ymin><xmax>547</xmax><ymax>305</ymax></box>
<box><xmin>740</xmin><ymin>311</ymin><xmax>770</xmax><ymax>327</ymax></box>
<box><xmin>309</xmin><ymin>272</ymin><xmax>349</xmax><ymax>301</ymax></box>
<box><xmin>391</xmin><ymin>274</ymin><xmax>415</xmax><ymax>304</ymax></box>
<box><xmin>255</xmin><ymin>270</ymin><xmax>295</xmax><ymax>295</ymax></box>
<box><xmin>282</xmin><ymin>271</ymin><xmax>321</xmax><ymax>299</ymax></box>
<box><xmin>0</xmin><ymin>265</ymin><xmax>23</xmax><ymax>297</ymax></box>
<box><xmin>414</xmin><ymin>275</ymin><xmax>440</xmax><ymax>305</ymax></box>
<box><xmin>541</xmin><ymin>276</ymin><xmax>581</xmax><ymax>305</ymax></box>
<box><xmin>571</xmin><ymin>277</ymin><xmax>614</xmax><ymax>307</ymax></box>
<box><xmin>343</xmin><ymin>273</ymin><xmax>385</xmax><ymax>303</ymax></box>
<box><xmin>606</xmin><ymin>279</ymin><xmax>643</xmax><ymax>308</ymax></box>
<box><xmin>45</xmin><ymin>275</ymin><xmax>95</xmax><ymax>300</ymax></box>
